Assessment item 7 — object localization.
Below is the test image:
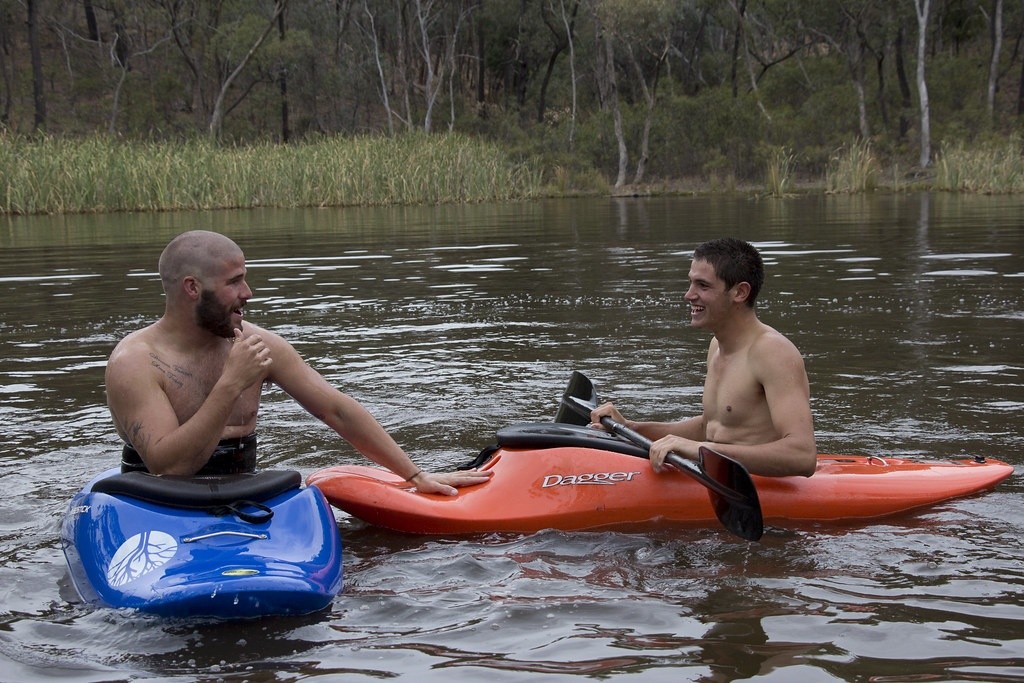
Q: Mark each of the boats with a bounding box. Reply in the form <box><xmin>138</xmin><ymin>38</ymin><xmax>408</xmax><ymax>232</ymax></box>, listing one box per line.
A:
<box><xmin>306</xmin><ymin>421</ymin><xmax>1016</xmax><ymax>536</ymax></box>
<box><xmin>57</xmin><ymin>464</ymin><xmax>342</xmax><ymax>625</ymax></box>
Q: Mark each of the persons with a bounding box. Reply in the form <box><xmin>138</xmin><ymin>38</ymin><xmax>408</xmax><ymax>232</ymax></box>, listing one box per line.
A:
<box><xmin>105</xmin><ymin>230</ymin><xmax>492</xmax><ymax>495</ymax></box>
<box><xmin>591</xmin><ymin>237</ymin><xmax>817</xmax><ymax>478</ymax></box>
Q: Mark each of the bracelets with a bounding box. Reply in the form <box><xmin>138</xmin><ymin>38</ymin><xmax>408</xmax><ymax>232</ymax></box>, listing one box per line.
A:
<box><xmin>406</xmin><ymin>469</ymin><xmax>421</xmax><ymax>482</ymax></box>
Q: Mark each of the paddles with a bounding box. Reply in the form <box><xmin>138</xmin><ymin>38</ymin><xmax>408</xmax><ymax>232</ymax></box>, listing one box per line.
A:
<box><xmin>552</xmin><ymin>369</ymin><xmax>764</xmax><ymax>543</ymax></box>
